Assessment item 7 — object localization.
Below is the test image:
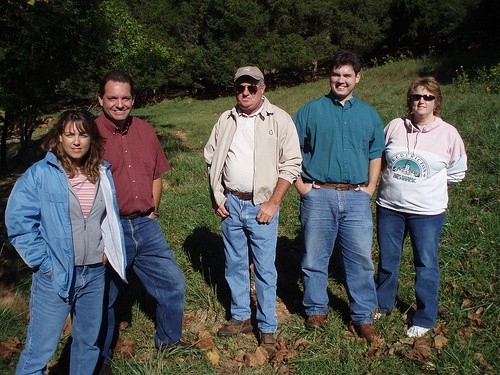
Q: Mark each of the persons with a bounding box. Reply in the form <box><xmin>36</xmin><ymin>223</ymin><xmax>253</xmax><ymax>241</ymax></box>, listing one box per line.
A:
<box><xmin>375</xmin><ymin>77</ymin><xmax>468</xmax><ymax>337</ymax></box>
<box><xmin>92</xmin><ymin>69</ymin><xmax>192</xmax><ymax>375</ymax></box>
<box><xmin>5</xmin><ymin>108</ymin><xmax>129</xmax><ymax>375</ymax></box>
<box><xmin>292</xmin><ymin>51</ymin><xmax>385</xmax><ymax>343</ymax></box>
<box><xmin>203</xmin><ymin>66</ymin><xmax>303</xmax><ymax>346</ymax></box>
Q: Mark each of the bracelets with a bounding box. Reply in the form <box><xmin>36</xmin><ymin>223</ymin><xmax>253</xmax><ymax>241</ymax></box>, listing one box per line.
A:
<box><xmin>152</xmin><ymin>210</ymin><xmax>160</xmax><ymax>216</ymax></box>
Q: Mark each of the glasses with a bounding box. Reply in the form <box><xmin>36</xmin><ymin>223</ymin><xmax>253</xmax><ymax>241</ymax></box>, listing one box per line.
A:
<box><xmin>234</xmin><ymin>85</ymin><xmax>262</xmax><ymax>94</ymax></box>
<box><xmin>410</xmin><ymin>94</ymin><xmax>435</xmax><ymax>101</ymax></box>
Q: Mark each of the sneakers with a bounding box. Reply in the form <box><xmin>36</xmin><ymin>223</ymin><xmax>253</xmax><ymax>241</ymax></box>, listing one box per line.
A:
<box><xmin>157</xmin><ymin>338</ymin><xmax>199</xmax><ymax>355</ymax></box>
<box><xmin>356</xmin><ymin>318</ymin><xmax>382</xmax><ymax>341</ymax></box>
<box><xmin>405</xmin><ymin>325</ymin><xmax>432</xmax><ymax>340</ymax></box>
<box><xmin>216</xmin><ymin>318</ymin><xmax>252</xmax><ymax>338</ymax></box>
<box><xmin>258</xmin><ymin>334</ymin><xmax>279</xmax><ymax>360</ymax></box>
<box><xmin>306</xmin><ymin>311</ymin><xmax>330</xmax><ymax>332</ymax></box>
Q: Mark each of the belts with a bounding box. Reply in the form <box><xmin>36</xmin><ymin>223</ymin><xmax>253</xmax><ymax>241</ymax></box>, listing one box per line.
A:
<box><xmin>120</xmin><ymin>209</ymin><xmax>155</xmax><ymax>220</ymax></box>
<box><xmin>308</xmin><ymin>180</ymin><xmax>365</xmax><ymax>191</ymax></box>
<box><xmin>227</xmin><ymin>189</ymin><xmax>253</xmax><ymax>201</ymax></box>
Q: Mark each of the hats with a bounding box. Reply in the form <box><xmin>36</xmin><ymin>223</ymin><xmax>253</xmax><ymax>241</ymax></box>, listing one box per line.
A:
<box><xmin>234</xmin><ymin>66</ymin><xmax>264</xmax><ymax>82</ymax></box>
